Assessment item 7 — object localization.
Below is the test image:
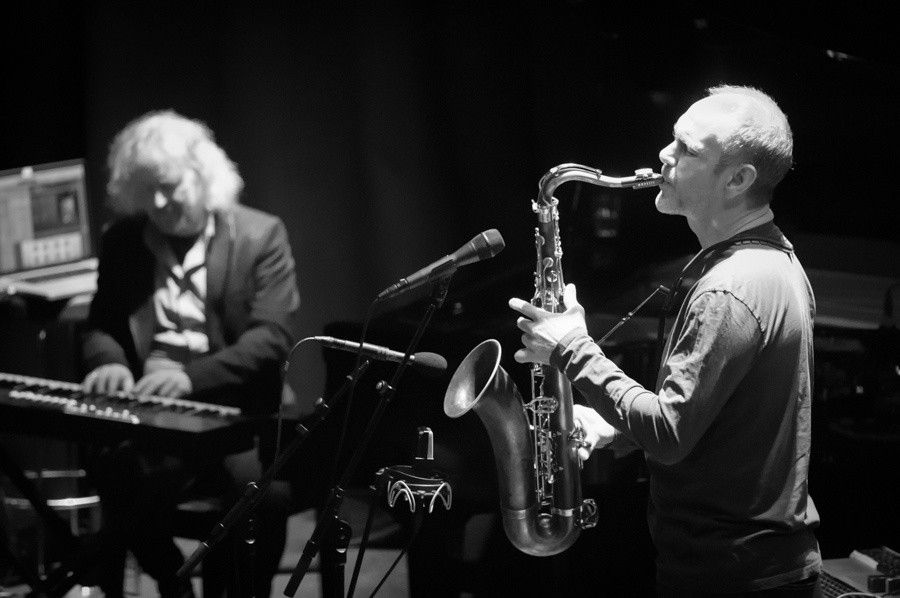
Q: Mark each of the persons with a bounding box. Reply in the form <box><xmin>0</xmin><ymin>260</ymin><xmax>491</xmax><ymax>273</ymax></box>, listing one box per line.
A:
<box><xmin>72</xmin><ymin>109</ymin><xmax>301</xmax><ymax>598</ymax></box>
<box><xmin>505</xmin><ymin>84</ymin><xmax>825</xmax><ymax>597</ymax></box>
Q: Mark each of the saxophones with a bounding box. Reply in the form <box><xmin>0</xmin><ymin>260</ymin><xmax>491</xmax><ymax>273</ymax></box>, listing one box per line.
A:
<box><xmin>444</xmin><ymin>161</ymin><xmax>667</xmax><ymax>557</ymax></box>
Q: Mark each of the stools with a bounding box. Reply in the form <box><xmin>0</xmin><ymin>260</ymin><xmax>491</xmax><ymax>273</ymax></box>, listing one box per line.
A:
<box><xmin>122</xmin><ymin>479</ymin><xmax>290</xmax><ymax>598</ymax></box>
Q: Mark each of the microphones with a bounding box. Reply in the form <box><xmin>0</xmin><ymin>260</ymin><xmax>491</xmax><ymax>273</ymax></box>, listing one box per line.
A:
<box><xmin>314</xmin><ymin>336</ymin><xmax>448</xmax><ymax>377</ymax></box>
<box><xmin>378</xmin><ymin>228</ymin><xmax>505</xmax><ymax>302</ymax></box>
<box><xmin>411</xmin><ymin>427</ymin><xmax>434</xmax><ymax>533</ymax></box>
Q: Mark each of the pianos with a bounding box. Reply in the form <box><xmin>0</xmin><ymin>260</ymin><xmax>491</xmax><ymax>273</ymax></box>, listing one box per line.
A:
<box><xmin>0</xmin><ymin>370</ymin><xmax>255</xmax><ymax>598</ymax></box>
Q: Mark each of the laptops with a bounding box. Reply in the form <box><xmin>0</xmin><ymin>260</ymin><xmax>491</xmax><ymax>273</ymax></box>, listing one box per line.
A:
<box><xmin>0</xmin><ymin>158</ymin><xmax>100</xmax><ymax>300</ymax></box>
